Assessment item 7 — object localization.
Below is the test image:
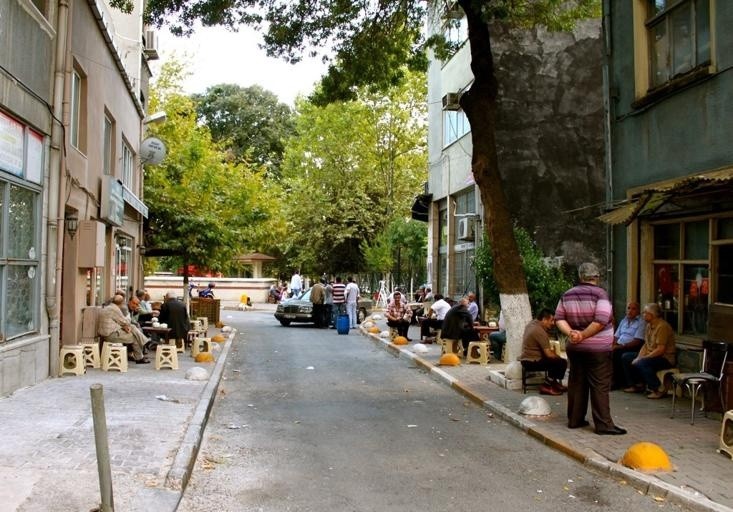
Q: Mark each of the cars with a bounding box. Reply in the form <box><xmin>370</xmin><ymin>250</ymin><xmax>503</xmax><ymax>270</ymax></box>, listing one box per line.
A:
<box><xmin>274</xmin><ymin>284</ymin><xmax>373</xmax><ymax>328</ymax></box>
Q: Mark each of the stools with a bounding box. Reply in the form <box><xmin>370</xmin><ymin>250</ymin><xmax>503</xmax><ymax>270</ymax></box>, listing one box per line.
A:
<box><xmin>549</xmin><ymin>340</ymin><xmax>561</xmax><ymax>357</ymax></box>
<box><xmin>716</xmin><ymin>409</ymin><xmax>733</xmax><ymax>461</ymax></box>
<box><xmin>655</xmin><ymin>368</ymin><xmax>682</xmax><ymax>399</ymax></box>
<box><xmin>389</xmin><ymin>314</ymin><xmax>509</xmax><ymax>366</ymax></box>
<box><xmin>58</xmin><ymin>314</ymin><xmax>211</xmax><ymax>377</ymax></box>
<box><xmin>521</xmin><ymin>365</ymin><xmax>547</xmax><ymax>395</ymax></box>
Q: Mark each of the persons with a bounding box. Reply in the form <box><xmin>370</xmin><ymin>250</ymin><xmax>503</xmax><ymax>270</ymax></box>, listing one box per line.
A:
<box><xmin>383</xmin><ymin>285</ymin><xmax>481</xmax><ymax>356</ymax></box>
<box><xmin>198</xmin><ymin>281</ymin><xmax>215</xmax><ymax>298</ymax></box>
<box><xmin>157</xmin><ymin>289</ymin><xmax>191</xmax><ymax>349</ymax></box>
<box><xmin>329</xmin><ymin>276</ymin><xmax>345</xmax><ymax>329</ymax></box>
<box><xmin>321</xmin><ymin>280</ymin><xmax>333</xmax><ymax>327</ymax></box>
<box><xmin>187</xmin><ymin>280</ymin><xmax>197</xmax><ymax>297</ymax></box>
<box><xmin>238</xmin><ymin>291</ymin><xmax>247</xmax><ymax>311</ymax></box>
<box><xmin>622</xmin><ymin>302</ymin><xmax>675</xmax><ymax>399</ymax></box>
<box><xmin>96</xmin><ymin>288</ymin><xmax>159</xmax><ymax>364</ymax></box>
<box><xmin>520</xmin><ymin>306</ymin><xmax>568</xmax><ymax>395</ymax></box>
<box><xmin>343</xmin><ymin>276</ymin><xmax>359</xmax><ymax>329</ymax></box>
<box><xmin>309</xmin><ymin>277</ymin><xmax>325</xmax><ymax>328</ymax></box>
<box><xmin>554</xmin><ymin>262</ymin><xmax>627</xmax><ymax>435</ymax></box>
<box><xmin>609</xmin><ymin>301</ymin><xmax>648</xmax><ymax>391</ymax></box>
<box><xmin>269</xmin><ymin>270</ymin><xmax>356</xmax><ymax>304</ymax></box>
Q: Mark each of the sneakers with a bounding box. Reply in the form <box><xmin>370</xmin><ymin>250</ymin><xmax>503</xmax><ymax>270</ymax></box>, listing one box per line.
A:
<box><xmin>136</xmin><ymin>357</ymin><xmax>150</xmax><ymax>363</ymax></box>
<box><xmin>148</xmin><ymin>341</ymin><xmax>159</xmax><ymax>350</ymax></box>
<box><xmin>143</xmin><ymin>347</ymin><xmax>148</xmax><ymax>355</ymax></box>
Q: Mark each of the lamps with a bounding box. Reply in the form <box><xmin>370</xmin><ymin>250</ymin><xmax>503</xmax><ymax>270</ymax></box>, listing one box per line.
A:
<box><xmin>64</xmin><ymin>214</ymin><xmax>78</xmax><ymax>241</ymax></box>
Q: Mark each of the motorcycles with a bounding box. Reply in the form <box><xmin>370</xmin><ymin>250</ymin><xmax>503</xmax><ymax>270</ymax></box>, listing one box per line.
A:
<box><xmin>189</xmin><ymin>279</ymin><xmax>215</xmax><ymax>300</ymax></box>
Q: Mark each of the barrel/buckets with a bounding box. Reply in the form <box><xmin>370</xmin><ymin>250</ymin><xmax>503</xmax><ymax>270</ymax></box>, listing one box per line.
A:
<box><xmin>336</xmin><ymin>314</ymin><xmax>350</xmax><ymax>334</ymax></box>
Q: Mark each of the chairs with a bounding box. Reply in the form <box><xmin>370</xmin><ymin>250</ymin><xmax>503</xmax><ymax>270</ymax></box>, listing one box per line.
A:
<box><xmin>670</xmin><ymin>339</ymin><xmax>733</xmax><ymax>425</ymax></box>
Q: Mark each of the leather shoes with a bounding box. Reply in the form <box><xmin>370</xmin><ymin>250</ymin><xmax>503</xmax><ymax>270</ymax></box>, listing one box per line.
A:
<box><xmin>568</xmin><ymin>422</ymin><xmax>589</xmax><ymax>428</ymax></box>
<box><xmin>596</xmin><ymin>426</ymin><xmax>627</xmax><ymax>434</ymax></box>
<box><xmin>553</xmin><ymin>382</ymin><xmax>568</xmax><ymax>392</ymax></box>
<box><xmin>538</xmin><ymin>383</ymin><xmax>563</xmax><ymax>395</ymax></box>
<box><xmin>647</xmin><ymin>385</ymin><xmax>668</xmax><ymax>399</ymax></box>
<box><xmin>623</xmin><ymin>383</ymin><xmax>646</xmax><ymax>393</ymax></box>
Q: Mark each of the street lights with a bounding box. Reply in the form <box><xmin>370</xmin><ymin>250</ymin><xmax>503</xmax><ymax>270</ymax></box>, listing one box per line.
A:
<box><xmin>395</xmin><ymin>240</ymin><xmax>403</xmax><ymax>287</ymax></box>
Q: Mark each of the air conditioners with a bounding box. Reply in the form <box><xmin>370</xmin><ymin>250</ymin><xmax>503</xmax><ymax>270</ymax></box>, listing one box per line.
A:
<box><xmin>456</xmin><ymin>216</ymin><xmax>475</xmax><ymax>242</ymax></box>
<box><xmin>144</xmin><ymin>30</ymin><xmax>160</xmax><ymax>60</ymax></box>
